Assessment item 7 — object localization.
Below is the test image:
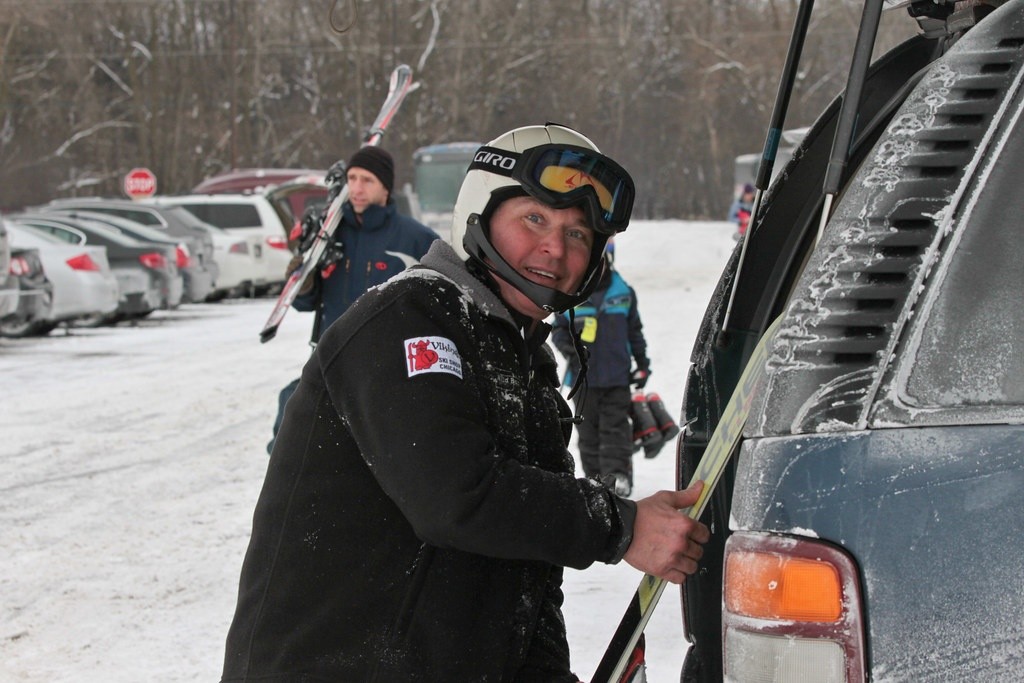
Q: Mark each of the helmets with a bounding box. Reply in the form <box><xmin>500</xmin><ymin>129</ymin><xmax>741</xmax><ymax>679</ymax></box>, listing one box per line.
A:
<box><xmin>450</xmin><ymin>121</ymin><xmax>615</xmax><ymax>312</ymax></box>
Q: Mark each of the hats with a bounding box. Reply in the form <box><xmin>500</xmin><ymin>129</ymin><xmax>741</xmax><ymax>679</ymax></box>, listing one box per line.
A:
<box><xmin>345</xmin><ymin>145</ymin><xmax>395</xmax><ymax>194</ymax></box>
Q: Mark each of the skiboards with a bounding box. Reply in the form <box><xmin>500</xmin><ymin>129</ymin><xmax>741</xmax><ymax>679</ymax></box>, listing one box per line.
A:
<box><xmin>259</xmin><ymin>63</ymin><xmax>423</xmax><ymax>346</ymax></box>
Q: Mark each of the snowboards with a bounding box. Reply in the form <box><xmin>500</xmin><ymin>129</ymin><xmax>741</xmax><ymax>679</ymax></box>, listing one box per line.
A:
<box><xmin>589</xmin><ymin>310</ymin><xmax>790</xmax><ymax>683</ymax></box>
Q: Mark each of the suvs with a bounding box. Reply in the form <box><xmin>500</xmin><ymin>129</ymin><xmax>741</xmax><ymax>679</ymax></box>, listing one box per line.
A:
<box><xmin>677</xmin><ymin>0</ymin><xmax>1024</xmax><ymax>683</ymax></box>
<box><xmin>47</xmin><ymin>169</ymin><xmax>328</xmax><ymax>304</ymax></box>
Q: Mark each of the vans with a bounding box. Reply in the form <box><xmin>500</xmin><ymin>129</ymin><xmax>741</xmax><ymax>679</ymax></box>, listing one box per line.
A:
<box><xmin>404</xmin><ymin>142</ymin><xmax>486</xmax><ymax>247</ymax></box>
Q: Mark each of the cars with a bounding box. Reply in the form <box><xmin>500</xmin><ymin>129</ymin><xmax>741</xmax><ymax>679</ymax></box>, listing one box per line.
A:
<box><xmin>0</xmin><ymin>169</ymin><xmax>182</xmax><ymax>338</ymax></box>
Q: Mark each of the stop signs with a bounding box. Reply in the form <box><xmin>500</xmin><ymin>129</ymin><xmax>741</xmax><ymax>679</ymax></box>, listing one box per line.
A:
<box><xmin>124</xmin><ymin>168</ymin><xmax>158</xmax><ymax>200</ymax></box>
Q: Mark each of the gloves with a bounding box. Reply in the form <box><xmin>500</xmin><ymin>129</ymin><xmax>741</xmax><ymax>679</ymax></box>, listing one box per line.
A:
<box><xmin>285</xmin><ymin>256</ymin><xmax>316</xmax><ymax>296</ymax></box>
<box><xmin>562</xmin><ymin>344</ymin><xmax>577</xmax><ymax>363</ymax></box>
<box><xmin>635</xmin><ymin>371</ymin><xmax>652</xmax><ymax>389</ymax></box>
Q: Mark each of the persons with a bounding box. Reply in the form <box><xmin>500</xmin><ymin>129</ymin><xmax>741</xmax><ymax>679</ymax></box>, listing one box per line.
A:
<box><xmin>730</xmin><ymin>183</ymin><xmax>758</xmax><ymax>240</ymax></box>
<box><xmin>286</xmin><ymin>144</ymin><xmax>449</xmax><ymax>353</ymax></box>
<box><xmin>222</xmin><ymin>121</ymin><xmax>711</xmax><ymax>683</ymax></box>
<box><xmin>545</xmin><ymin>234</ymin><xmax>652</xmax><ymax>503</ymax></box>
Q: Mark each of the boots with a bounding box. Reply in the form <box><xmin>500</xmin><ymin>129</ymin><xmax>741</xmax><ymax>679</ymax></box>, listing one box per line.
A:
<box><xmin>628</xmin><ymin>392</ymin><xmax>662</xmax><ymax>454</ymax></box>
<box><xmin>644</xmin><ymin>393</ymin><xmax>679</xmax><ymax>458</ymax></box>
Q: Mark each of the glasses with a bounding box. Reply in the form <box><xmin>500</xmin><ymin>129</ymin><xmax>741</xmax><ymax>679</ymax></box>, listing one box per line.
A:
<box><xmin>466</xmin><ymin>143</ymin><xmax>635</xmax><ymax>237</ymax></box>
